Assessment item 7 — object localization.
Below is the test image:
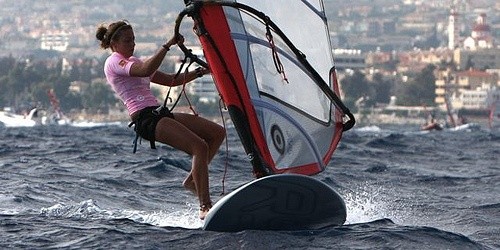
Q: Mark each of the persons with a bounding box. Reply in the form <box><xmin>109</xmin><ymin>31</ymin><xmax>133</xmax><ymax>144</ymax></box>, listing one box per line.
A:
<box><xmin>95</xmin><ymin>18</ymin><xmax>229</xmax><ymax>219</ymax></box>
